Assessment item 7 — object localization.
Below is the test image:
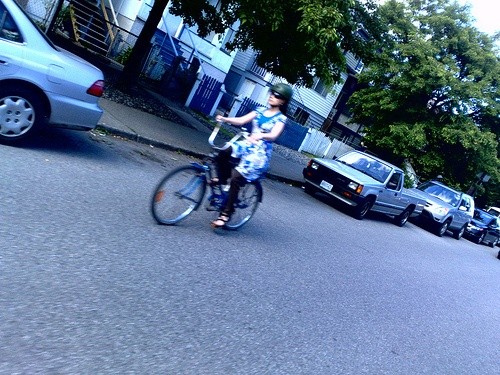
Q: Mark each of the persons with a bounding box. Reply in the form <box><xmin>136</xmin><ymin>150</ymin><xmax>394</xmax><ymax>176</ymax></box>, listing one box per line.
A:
<box><xmin>206</xmin><ymin>83</ymin><xmax>292</xmax><ymax>227</ymax></box>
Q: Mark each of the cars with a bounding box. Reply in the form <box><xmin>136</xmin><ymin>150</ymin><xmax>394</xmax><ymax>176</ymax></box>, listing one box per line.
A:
<box><xmin>0</xmin><ymin>0</ymin><xmax>105</xmax><ymax>144</ymax></box>
<box><xmin>464</xmin><ymin>207</ymin><xmax>500</xmax><ymax>248</ymax></box>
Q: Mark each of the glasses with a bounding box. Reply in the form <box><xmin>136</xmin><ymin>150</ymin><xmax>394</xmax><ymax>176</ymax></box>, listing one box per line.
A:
<box><xmin>270</xmin><ymin>90</ymin><xmax>286</xmax><ymax>101</ymax></box>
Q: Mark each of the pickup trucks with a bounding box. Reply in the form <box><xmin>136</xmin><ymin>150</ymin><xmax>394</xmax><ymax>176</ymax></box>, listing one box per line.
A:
<box><xmin>302</xmin><ymin>149</ymin><xmax>427</xmax><ymax>227</ymax></box>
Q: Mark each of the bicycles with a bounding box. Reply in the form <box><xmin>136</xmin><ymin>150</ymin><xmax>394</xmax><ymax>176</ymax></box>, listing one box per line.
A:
<box><xmin>151</xmin><ymin>115</ymin><xmax>263</xmax><ymax>231</ymax></box>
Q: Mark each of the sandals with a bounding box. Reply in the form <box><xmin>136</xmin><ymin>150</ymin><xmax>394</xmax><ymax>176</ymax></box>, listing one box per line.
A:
<box><xmin>211</xmin><ymin>213</ymin><xmax>231</xmax><ymax>227</ymax></box>
<box><xmin>207</xmin><ymin>178</ymin><xmax>228</xmax><ymax>186</ymax></box>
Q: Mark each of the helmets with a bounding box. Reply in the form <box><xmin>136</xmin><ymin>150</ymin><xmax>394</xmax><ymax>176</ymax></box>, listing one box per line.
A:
<box><xmin>269</xmin><ymin>83</ymin><xmax>293</xmax><ymax>105</ymax></box>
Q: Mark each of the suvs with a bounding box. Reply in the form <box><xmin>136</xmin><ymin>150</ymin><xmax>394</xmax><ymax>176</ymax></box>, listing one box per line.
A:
<box><xmin>409</xmin><ymin>180</ymin><xmax>475</xmax><ymax>240</ymax></box>
